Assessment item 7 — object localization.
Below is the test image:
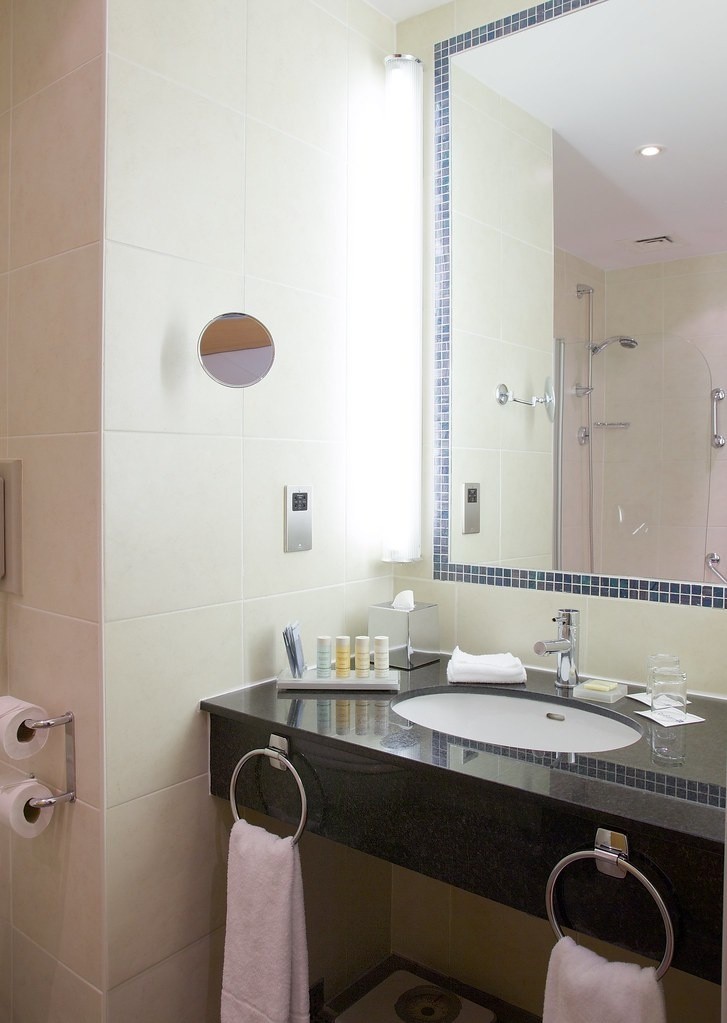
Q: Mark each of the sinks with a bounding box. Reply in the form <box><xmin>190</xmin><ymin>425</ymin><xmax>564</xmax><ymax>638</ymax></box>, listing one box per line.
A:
<box><xmin>389</xmin><ymin>682</ymin><xmax>648</xmax><ymax>756</ymax></box>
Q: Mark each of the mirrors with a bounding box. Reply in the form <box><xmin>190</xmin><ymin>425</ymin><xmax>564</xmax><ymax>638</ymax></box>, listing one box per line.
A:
<box><xmin>429</xmin><ymin>0</ymin><xmax>724</xmax><ymax>616</ymax></box>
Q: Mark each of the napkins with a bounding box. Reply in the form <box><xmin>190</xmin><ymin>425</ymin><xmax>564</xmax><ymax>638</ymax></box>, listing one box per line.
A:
<box><xmin>368</xmin><ymin>590</ymin><xmax>441</xmax><ymax>671</ymax></box>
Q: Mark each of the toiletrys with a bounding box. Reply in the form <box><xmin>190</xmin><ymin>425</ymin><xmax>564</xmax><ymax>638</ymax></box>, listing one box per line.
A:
<box><xmin>312</xmin><ymin>697</ymin><xmax>334</xmax><ymax>736</ymax></box>
<box><xmin>333</xmin><ymin>635</ymin><xmax>353</xmax><ymax>677</ymax></box>
<box><xmin>314</xmin><ymin>635</ymin><xmax>334</xmax><ymax>677</ymax></box>
<box><xmin>333</xmin><ymin>699</ymin><xmax>353</xmax><ymax>736</ymax></box>
<box><xmin>353</xmin><ymin>634</ymin><xmax>371</xmax><ymax>676</ymax></box>
<box><xmin>374</xmin><ymin>699</ymin><xmax>390</xmax><ymax>737</ymax></box>
<box><xmin>373</xmin><ymin>634</ymin><xmax>393</xmax><ymax>677</ymax></box>
<box><xmin>354</xmin><ymin>698</ymin><xmax>370</xmax><ymax>737</ymax></box>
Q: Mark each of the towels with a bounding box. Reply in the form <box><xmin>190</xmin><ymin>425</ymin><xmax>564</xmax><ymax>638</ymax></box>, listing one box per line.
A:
<box><xmin>538</xmin><ymin>937</ymin><xmax>669</xmax><ymax>1023</ymax></box>
<box><xmin>219</xmin><ymin>817</ymin><xmax>313</xmax><ymax>1023</ymax></box>
<box><xmin>444</xmin><ymin>645</ymin><xmax>529</xmax><ymax>685</ymax></box>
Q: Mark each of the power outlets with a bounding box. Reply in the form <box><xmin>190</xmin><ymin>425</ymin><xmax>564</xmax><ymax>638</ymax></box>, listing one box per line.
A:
<box><xmin>283</xmin><ymin>485</ymin><xmax>313</xmax><ymax>553</ymax></box>
<box><xmin>461</xmin><ymin>482</ymin><xmax>481</xmax><ymax>535</ymax></box>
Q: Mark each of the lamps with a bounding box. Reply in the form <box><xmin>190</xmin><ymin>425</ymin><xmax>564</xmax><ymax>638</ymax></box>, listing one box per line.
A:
<box><xmin>382</xmin><ymin>55</ymin><xmax>422</xmax><ymax>569</ymax></box>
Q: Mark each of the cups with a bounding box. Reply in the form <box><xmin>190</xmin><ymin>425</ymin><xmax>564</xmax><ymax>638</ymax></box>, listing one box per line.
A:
<box><xmin>646</xmin><ymin>652</ymin><xmax>688</xmax><ymax>724</ymax></box>
<box><xmin>652</xmin><ymin>667</ymin><xmax>690</xmax><ymax>765</ymax></box>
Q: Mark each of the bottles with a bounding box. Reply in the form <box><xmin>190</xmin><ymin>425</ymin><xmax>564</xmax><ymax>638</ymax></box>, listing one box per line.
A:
<box><xmin>354</xmin><ymin>635</ymin><xmax>370</xmax><ymax>678</ymax></box>
<box><xmin>316</xmin><ymin>636</ymin><xmax>332</xmax><ymax>678</ymax></box>
<box><xmin>335</xmin><ymin>636</ymin><xmax>350</xmax><ymax>678</ymax></box>
<box><xmin>374</xmin><ymin>636</ymin><xmax>389</xmax><ymax>679</ymax></box>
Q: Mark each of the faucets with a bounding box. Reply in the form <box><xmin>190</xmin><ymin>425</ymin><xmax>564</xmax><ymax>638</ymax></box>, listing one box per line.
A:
<box><xmin>532</xmin><ymin>608</ymin><xmax>584</xmax><ymax>688</ymax></box>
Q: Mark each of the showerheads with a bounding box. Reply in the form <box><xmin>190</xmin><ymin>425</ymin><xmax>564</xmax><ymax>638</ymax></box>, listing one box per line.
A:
<box><xmin>592</xmin><ymin>334</ymin><xmax>640</xmax><ymax>356</ymax></box>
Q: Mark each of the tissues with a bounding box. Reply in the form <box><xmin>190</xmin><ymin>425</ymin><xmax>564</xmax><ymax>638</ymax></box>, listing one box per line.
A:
<box><xmin>365</xmin><ymin>587</ymin><xmax>443</xmax><ymax>672</ymax></box>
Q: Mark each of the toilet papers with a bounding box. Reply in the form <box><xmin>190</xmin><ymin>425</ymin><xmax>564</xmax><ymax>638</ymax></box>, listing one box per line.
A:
<box><xmin>0</xmin><ymin>691</ymin><xmax>54</xmax><ymax>763</ymax></box>
<box><xmin>0</xmin><ymin>780</ymin><xmax>54</xmax><ymax>840</ymax></box>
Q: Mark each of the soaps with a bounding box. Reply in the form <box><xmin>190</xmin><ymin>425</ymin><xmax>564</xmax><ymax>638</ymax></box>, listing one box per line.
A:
<box><xmin>583</xmin><ymin>677</ymin><xmax>620</xmax><ymax>692</ymax></box>
